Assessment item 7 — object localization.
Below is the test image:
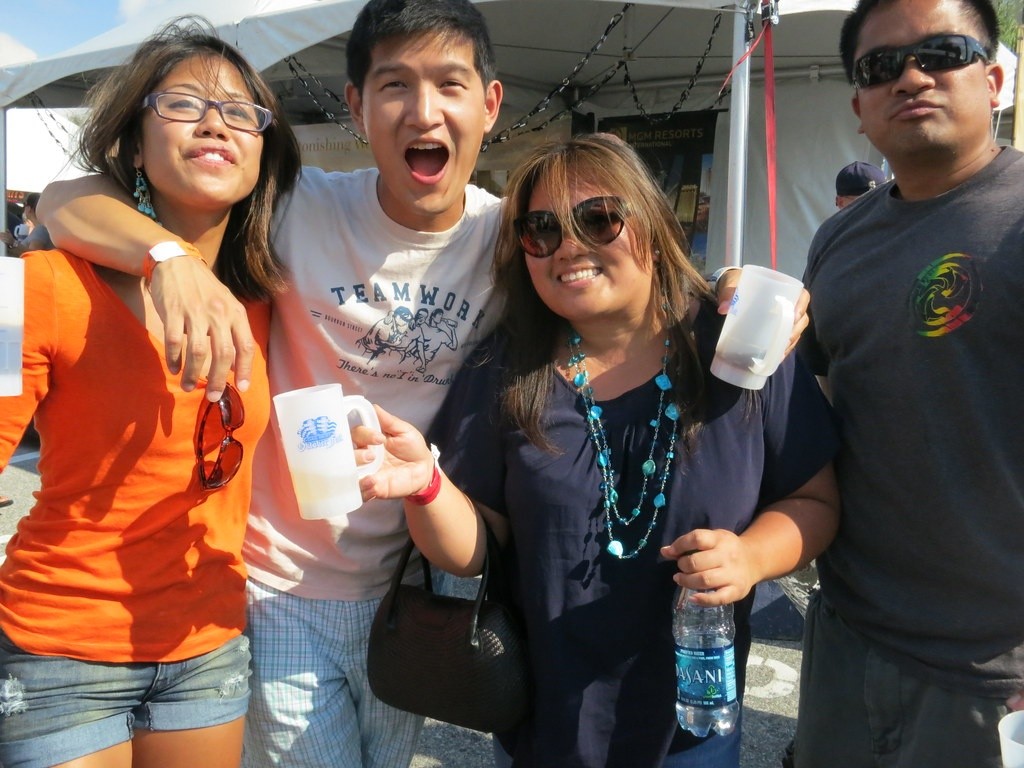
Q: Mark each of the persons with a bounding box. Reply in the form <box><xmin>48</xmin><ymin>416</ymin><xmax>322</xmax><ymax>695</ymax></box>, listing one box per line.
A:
<box><xmin>831</xmin><ymin>154</ymin><xmax>891</xmax><ymax>221</ymax></box>
<box><xmin>34</xmin><ymin>0</ymin><xmax>814</xmax><ymax>767</ymax></box>
<box><xmin>0</xmin><ymin>191</ymin><xmax>57</xmax><ymax>259</ymax></box>
<box><xmin>1</xmin><ymin>27</ymin><xmax>278</xmax><ymax>768</ymax></box>
<box><xmin>351</xmin><ymin>124</ymin><xmax>847</xmax><ymax>768</ymax></box>
<box><xmin>785</xmin><ymin>1</ymin><xmax>1023</xmax><ymax>767</ymax></box>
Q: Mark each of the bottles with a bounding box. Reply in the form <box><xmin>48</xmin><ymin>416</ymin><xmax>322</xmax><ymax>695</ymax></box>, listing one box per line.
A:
<box><xmin>673</xmin><ymin>586</ymin><xmax>739</xmax><ymax>738</ymax></box>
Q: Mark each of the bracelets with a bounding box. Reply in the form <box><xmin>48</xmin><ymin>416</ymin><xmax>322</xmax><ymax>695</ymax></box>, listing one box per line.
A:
<box><xmin>707</xmin><ymin>264</ymin><xmax>745</xmax><ymax>299</ymax></box>
<box><xmin>401</xmin><ymin>463</ymin><xmax>442</xmax><ymax>506</ymax></box>
<box><xmin>144</xmin><ymin>240</ymin><xmax>210</xmax><ymax>293</ymax></box>
<box><xmin>12</xmin><ymin>239</ymin><xmax>18</xmax><ymax>248</ymax></box>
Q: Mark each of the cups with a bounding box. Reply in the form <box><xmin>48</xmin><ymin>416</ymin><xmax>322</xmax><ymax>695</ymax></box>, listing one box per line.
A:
<box><xmin>14</xmin><ymin>224</ymin><xmax>30</xmax><ymax>242</ymax></box>
<box><xmin>710</xmin><ymin>265</ymin><xmax>804</xmax><ymax>390</ymax></box>
<box><xmin>998</xmin><ymin>710</ymin><xmax>1024</xmax><ymax>768</ymax></box>
<box><xmin>272</xmin><ymin>383</ymin><xmax>385</xmax><ymax>519</ymax></box>
<box><xmin>0</xmin><ymin>256</ymin><xmax>25</xmax><ymax>396</ymax></box>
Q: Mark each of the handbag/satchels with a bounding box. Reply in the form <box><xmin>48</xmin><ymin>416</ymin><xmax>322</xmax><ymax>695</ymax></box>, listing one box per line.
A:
<box><xmin>366</xmin><ymin>510</ymin><xmax>533</xmax><ymax>733</ymax></box>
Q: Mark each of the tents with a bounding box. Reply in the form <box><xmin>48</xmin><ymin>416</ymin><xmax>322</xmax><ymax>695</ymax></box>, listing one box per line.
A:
<box><xmin>3</xmin><ymin>0</ymin><xmax>1024</xmax><ymax>280</ymax></box>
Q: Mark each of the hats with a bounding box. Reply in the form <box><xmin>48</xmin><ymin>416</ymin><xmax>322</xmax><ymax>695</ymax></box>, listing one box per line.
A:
<box><xmin>835</xmin><ymin>161</ymin><xmax>885</xmax><ymax>206</ymax></box>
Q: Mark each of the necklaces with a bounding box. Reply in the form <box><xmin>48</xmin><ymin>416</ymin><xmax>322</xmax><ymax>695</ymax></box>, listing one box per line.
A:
<box><xmin>560</xmin><ymin>280</ymin><xmax>687</xmax><ymax>560</ymax></box>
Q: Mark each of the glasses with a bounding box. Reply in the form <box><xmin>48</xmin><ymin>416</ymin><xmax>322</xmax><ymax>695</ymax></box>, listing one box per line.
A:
<box><xmin>199</xmin><ymin>383</ymin><xmax>246</xmax><ymax>487</ymax></box>
<box><xmin>136</xmin><ymin>92</ymin><xmax>274</xmax><ymax>132</ymax></box>
<box><xmin>852</xmin><ymin>34</ymin><xmax>992</xmax><ymax>90</ymax></box>
<box><xmin>512</xmin><ymin>196</ymin><xmax>632</xmax><ymax>258</ymax></box>
